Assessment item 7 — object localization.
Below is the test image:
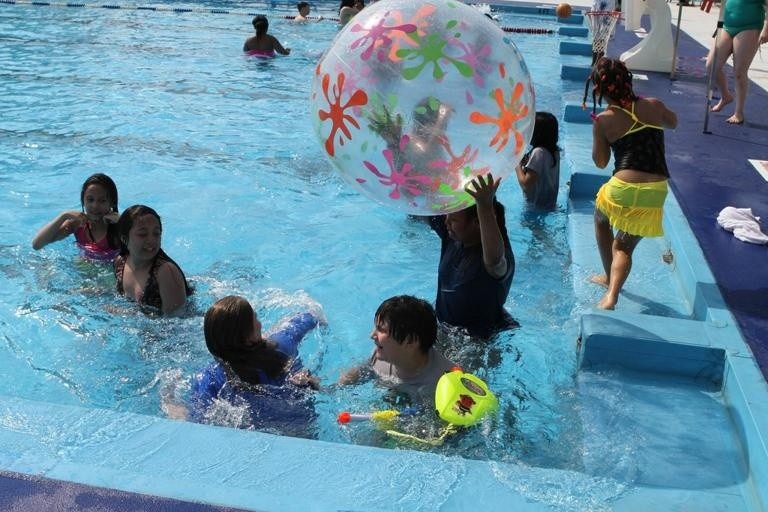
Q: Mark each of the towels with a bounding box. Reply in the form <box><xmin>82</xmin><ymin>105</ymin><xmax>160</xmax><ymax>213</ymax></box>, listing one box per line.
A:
<box><xmin>715</xmin><ymin>203</ymin><xmax>767</xmax><ymax>247</ymax></box>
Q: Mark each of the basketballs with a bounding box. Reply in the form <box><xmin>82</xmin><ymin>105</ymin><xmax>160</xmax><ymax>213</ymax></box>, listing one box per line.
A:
<box><xmin>556</xmin><ymin>3</ymin><xmax>572</xmax><ymax>18</ymax></box>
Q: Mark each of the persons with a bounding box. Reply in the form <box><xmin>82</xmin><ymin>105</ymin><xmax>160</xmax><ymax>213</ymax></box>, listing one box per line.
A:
<box><xmin>286</xmin><ymin>293</ymin><xmax>468</xmax><ymax>451</ymax></box>
<box><xmin>339</xmin><ymin>0</ymin><xmax>361</xmax><ymax>27</ymax></box>
<box><xmin>395</xmin><ymin>97</ymin><xmax>457</xmax><ymax>219</ymax></box>
<box><xmin>243</xmin><ymin>15</ymin><xmax>291</xmax><ymax>61</ymax></box>
<box><xmin>517</xmin><ymin>109</ymin><xmax>563</xmax><ymax>265</ymax></box>
<box><xmin>293</xmin><ymin>2</ymin><xmax>323</xmax><ymax>25</ymax></box>
<box><xmin>582</xmin><ymin>56</ymin><xmax>679</xmax><ymax>314</ymax></box>
<box><xmin>155</xmin><ymin>293</ymin><xmax>327</xmax><ymax>438</ymax></box>
<box><xmin>354</xmin><ymin>1</ymin><xmax>365</xmax><ymax>11</ymax></box>
<box><xmin>705</xmin><ymin>0</ymin><xmax>767</xmax><ymax>126</ymax></box>
<box><xmin>368</xmin><ymin>105</ymin><xmax>523</xmax><ymax>373</ymax></box>
<box><xmin>70</xmin><ymin>203</ymin><xmax>192</xmax><ymax>322</ymax></box>
<box><xmin>32</xmin><ymin>173</ymin><xmax>121</xmax><ymax>274</ymax></box>
